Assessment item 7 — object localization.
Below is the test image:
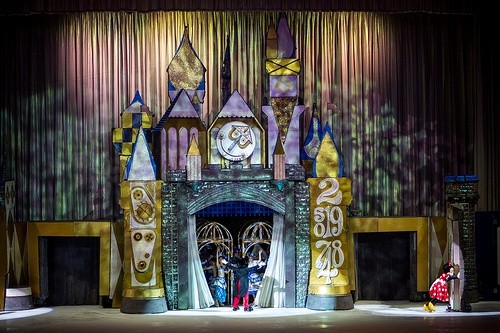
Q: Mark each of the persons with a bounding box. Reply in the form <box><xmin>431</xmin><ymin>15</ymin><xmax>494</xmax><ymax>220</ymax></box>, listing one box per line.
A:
<box><xmin>200</xmin><ymin>253</ymin><xmax>266</xmax><ymax>312</ymax></box>
